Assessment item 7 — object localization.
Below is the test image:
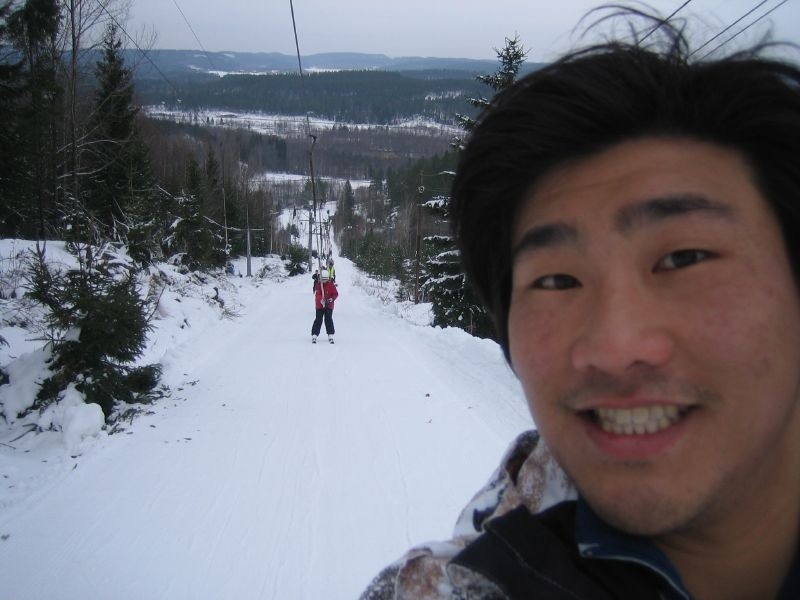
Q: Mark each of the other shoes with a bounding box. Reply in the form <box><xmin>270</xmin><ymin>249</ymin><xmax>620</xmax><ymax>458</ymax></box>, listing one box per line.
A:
<box><xmin>312</xmin><ymin>335</ymin><xmax>317</xmax><ymax>343</ymax></box>
<box><xmin>328</xmin><ymin>335</ymin><xmax>334</xmax><ymax>343</ymax></box>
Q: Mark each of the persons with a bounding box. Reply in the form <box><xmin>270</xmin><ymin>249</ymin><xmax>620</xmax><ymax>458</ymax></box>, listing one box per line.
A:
<box><xmin>311</xmin><ymin>260</ymin><xmax>338</xmax><ymax>344</ymax></box>
<box><xmin>360</xmin><ymin>5</ymin><xmax>800</xmax><ymax>600</ymax></box>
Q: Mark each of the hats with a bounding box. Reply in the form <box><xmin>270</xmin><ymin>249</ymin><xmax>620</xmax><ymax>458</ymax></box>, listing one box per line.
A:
<box><xmin>320</xmin><ymin>270</ymin><xmax>329</xmax><ymax>280</ymax></box>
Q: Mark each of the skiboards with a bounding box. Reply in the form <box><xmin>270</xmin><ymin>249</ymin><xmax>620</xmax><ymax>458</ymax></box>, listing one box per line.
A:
<box><xmin>311</xmin><ymin>338</ymin><xmax>334</xmax><ymax>344</ymax></box>
